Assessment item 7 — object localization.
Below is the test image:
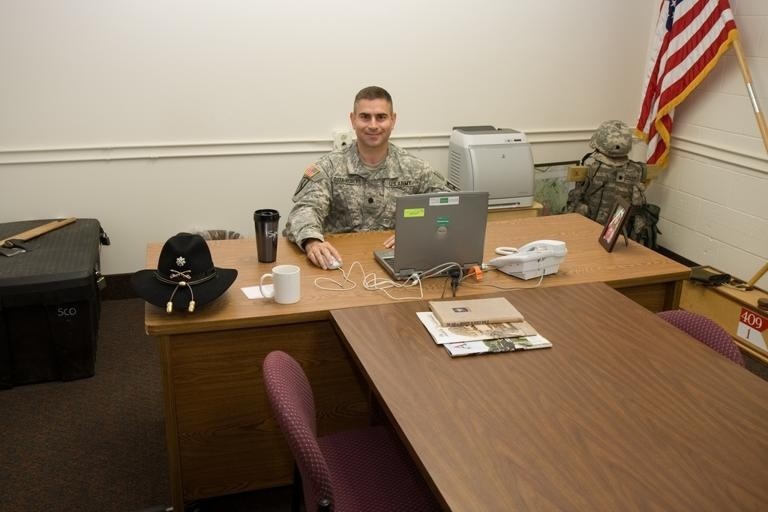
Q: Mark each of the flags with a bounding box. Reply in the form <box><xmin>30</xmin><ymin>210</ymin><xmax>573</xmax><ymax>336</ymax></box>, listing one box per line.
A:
<box><xmin>630</xmin><ymin>0</ymin><xmax>738</xmax><ymax>189</ymax></box>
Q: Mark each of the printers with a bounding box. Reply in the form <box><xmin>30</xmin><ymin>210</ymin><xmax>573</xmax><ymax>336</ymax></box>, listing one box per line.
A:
<box><xmin>449</xmin><ymin>124</ymin><xmax>535</xmax><ymax>209</ymax></box>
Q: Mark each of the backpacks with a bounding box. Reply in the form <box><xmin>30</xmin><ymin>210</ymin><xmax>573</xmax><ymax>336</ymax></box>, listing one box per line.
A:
<box><xmin>560</xmin><ymin>151</ymin><xmax>648</xmax><ymax>248</ymax></box>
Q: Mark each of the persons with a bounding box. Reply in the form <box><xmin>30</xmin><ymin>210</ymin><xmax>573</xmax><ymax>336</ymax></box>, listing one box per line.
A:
<box><xmin>566</xmin><ymin>121</ymin><xmax>646</xmax><ymax>234</ymax></box>
<box><xmin>283</xmin><ymin>86</ymin><xmax>448</xmax><ymax>270</ymax></box>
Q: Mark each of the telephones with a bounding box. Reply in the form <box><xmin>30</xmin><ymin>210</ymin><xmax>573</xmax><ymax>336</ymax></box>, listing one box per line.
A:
<box><xmin>488</xmin><ymin>240</ymin><xmax>567</xmax><ymax>280</ymax></box>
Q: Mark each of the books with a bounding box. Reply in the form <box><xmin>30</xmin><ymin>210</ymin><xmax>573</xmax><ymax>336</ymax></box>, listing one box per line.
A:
<box><xmin>416</xmin><ymin>297</ymin><xmax>552</xmax><ymax>357</ymax></box>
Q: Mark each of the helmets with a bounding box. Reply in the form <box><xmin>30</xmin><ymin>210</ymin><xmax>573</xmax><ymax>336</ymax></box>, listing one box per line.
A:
<box><xmin>590</xmin><ymin>120</ymin><xmax>632</xmax><ymax>157</ymax></box>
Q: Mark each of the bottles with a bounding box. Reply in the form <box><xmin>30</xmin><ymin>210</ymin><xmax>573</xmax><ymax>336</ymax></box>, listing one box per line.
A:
<box><xmin>254</xmin><ymin>209</ymin><xmax>280</xmax><ymax>262</ymax></box>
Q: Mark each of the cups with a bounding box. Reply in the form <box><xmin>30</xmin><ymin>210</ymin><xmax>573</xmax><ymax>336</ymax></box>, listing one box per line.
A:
<box><xmin>260</xmin><ymin>265</ymin><xmax>301</xmax><ymax>304</ymax></box>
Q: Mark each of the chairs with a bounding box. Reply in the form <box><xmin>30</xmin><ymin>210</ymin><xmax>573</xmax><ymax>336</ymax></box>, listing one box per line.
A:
<box><xmin>262</xmin><ymin>350</ymin><xmax>442</xmax><ymax>510</ymax></box>
<box><xmin>655</xmin><ymin>311</ymin><xmax>747</xmax><ymax>370</ymax></box>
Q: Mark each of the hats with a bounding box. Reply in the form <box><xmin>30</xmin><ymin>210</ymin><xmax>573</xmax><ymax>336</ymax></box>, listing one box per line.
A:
<box><xmin>130</xmin><ymin>233</ymin><xmax>238</xmax><ymax>311</ymax></box>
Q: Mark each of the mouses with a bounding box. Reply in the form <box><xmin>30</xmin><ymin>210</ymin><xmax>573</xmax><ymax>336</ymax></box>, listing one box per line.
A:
<box><xmin>322</xmin><ymin>255</ymin><xmax>341</xmax><ymax>270</ymax></box>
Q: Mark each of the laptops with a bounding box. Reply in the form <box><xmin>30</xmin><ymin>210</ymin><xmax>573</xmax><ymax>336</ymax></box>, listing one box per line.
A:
<box><xmin>373</xmin><ymin>191</ymin><xmax>490</xmax><ymax>282</ymax></box>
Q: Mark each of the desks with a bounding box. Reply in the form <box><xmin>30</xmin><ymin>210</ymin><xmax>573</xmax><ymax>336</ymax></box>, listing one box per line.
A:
<box><xmin>328</xmin><ymin>281</ymin><xmax>768</xmax><ymax>512</ymax></box>
<box><xmin>143</xmin><ymin>212</ymin><xmax>693</xmax><ymax>510</ymax></box>
